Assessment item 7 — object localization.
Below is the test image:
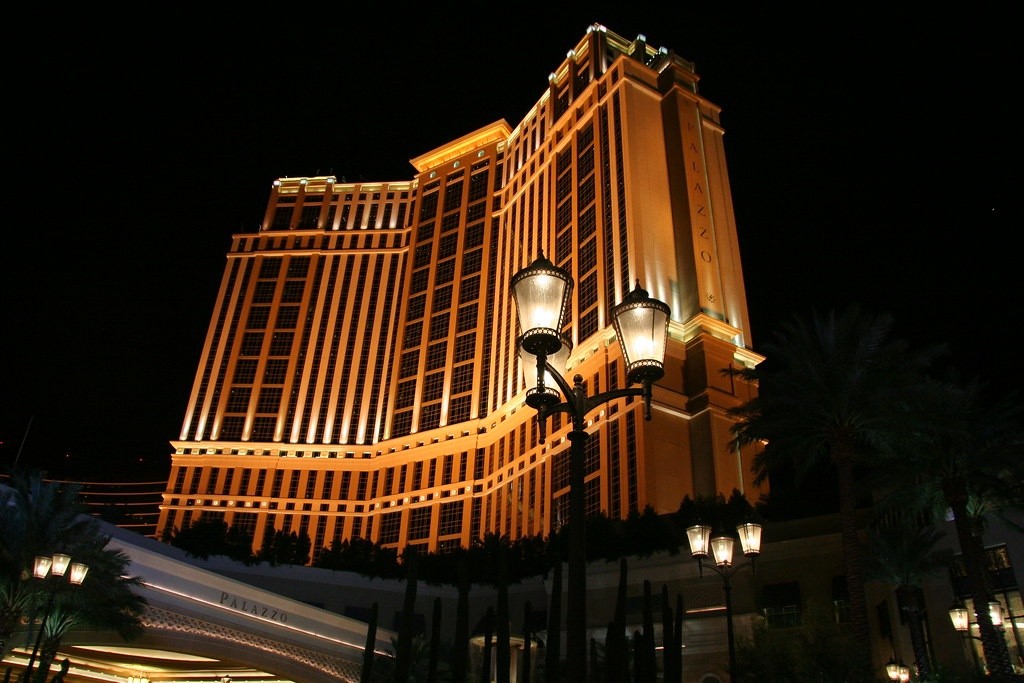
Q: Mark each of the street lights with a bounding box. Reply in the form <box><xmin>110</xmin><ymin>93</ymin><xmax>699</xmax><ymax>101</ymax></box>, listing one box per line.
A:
<box><xmin>23</xmin><ymin>552</ymin><xmax>90</xmax><ymax>679</ymax></box>
<box><xmin>510</xmin><ymin>245</ymin><xmax>671</xmax><ymax>683</ymax></box>
<box><xmin>686</xmin><ymin>516</ymin><xmax>763</xmax><ymax>683</ymax></box>
